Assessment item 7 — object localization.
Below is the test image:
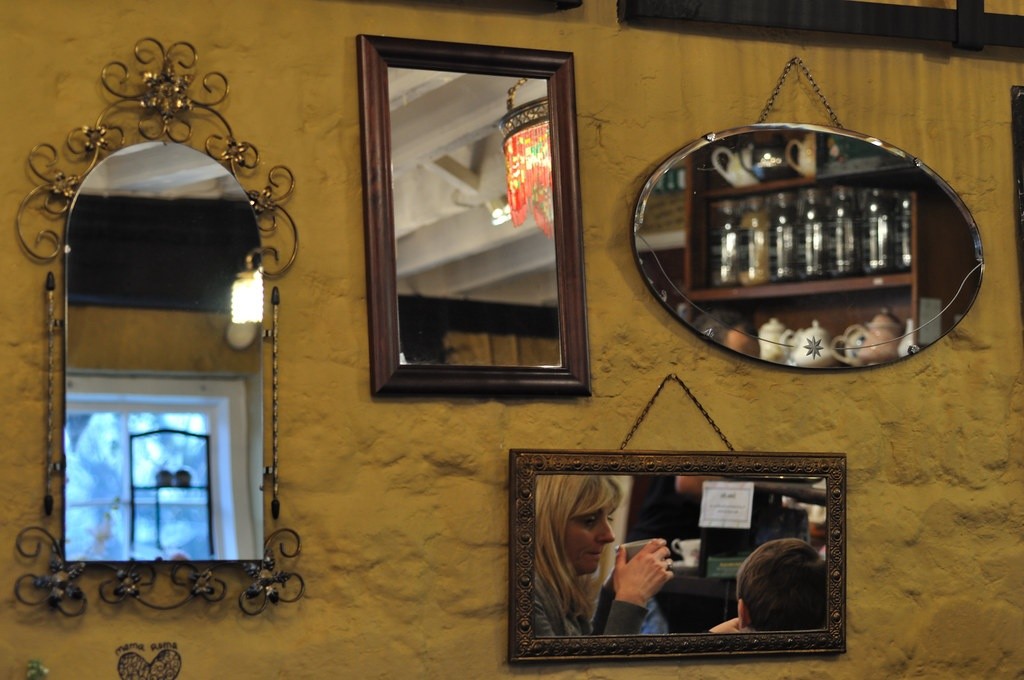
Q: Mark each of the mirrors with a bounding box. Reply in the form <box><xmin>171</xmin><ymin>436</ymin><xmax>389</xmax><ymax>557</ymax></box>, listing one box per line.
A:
<box><xmin>15</xmin><ymin>33</ymin><xmax>307</xmax><ymax>618</ymax></box>
<box><xmin>628</xmin><ymin>119</ymin><xmax>986</xmax><ymax>376</ymax></box>
<box><xmin>506</xmin><ymin>449</ymin><xmax>849</xmax><ymax>665</ymax></box>
<box><xmin>354</xmin><ymin>31</ymin><xmax>593</xmax><ymax>407</ymax></box>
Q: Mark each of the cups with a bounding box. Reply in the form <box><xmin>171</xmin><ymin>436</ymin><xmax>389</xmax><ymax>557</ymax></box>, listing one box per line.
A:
<box><xmin>671</xmin><ymin>539</ymin><xmax>701</xmax><ymax>568</ymax></box>
<box><xmin>752</xmin><ymin>148</ymin><xmax>789</xmax><ymax>180</ymax></box>
<box><xmin>615</xmin><ymin>539</ymin><xmax>666</xmax><ymax>563</ymax></box>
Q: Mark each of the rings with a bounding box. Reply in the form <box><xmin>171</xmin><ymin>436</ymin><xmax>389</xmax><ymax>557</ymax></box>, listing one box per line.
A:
<box><xmin>666</xmin><ymin>559</ymin><xmax>672</xmax><ymax>569</ymax></box>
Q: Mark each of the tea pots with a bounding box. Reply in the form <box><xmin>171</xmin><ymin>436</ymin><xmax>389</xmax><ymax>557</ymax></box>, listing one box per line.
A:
<box><xmin>711</xmin><ymin>143</ymin><xmax>760</xmax><ymax>187</ymax></box>
<box><xmin>724</xmin><ymin>307</ymin><xmax>901</xmax><ymax>368</ymax></box>
<box><xmin>786</xmin><ymin>133</ymin><xmax>816</xmax><ymax>178</ymax></box>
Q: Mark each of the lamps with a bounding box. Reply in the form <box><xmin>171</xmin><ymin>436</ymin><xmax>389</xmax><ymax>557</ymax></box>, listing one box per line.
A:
<box><xmin>494</xmin><ymin>79</ymin><xmax>555</xmax><ymax>244</ymax></box>
<box><xmin>230</xmin><ymin>254</ymin><xmax>264</xmax><ymax>324</ymax></box>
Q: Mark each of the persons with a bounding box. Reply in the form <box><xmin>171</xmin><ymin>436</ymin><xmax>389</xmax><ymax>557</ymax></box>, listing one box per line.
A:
<box><xmin>706</xmin><ymin>538</ymin><xmax>824</xmax><ymax>631</ymax></box>
<box><xmin>533</xmin><ymin>474</ymin><xmax>673</xmax><ymax>633</ymax></box>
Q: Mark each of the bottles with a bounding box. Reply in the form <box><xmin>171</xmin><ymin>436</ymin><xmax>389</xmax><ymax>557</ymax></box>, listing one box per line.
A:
<box><xmin>919</xmin><ymin>292</ymin><xmax>942</xmax><ymax>346</ymax></box>
<box><xmin>706</xmin><ymin>185</ymin><xmax>910</xmax><ymax>287</ymax></box>
<box><xmin>898</xmin><ymin>318</ymin><xmax>914</xmax><ymax>357</ymax></box>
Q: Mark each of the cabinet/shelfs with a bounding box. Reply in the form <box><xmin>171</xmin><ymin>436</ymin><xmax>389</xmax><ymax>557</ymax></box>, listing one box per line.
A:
<box><xmin>685</xmin><ymin>130</ymin><xmax>921</xmax><ymax>356</ymax></box>
